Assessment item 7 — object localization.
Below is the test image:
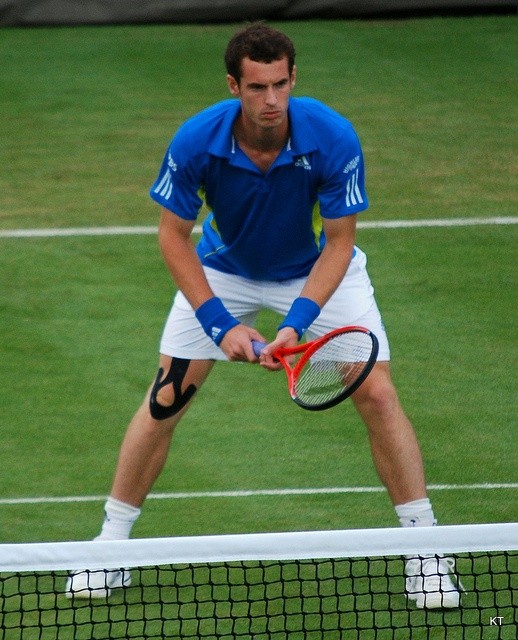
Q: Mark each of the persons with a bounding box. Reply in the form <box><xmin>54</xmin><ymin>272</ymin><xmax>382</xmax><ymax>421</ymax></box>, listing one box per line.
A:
<box><xmin>65</xmin><ymin>22</ymin><xmax>463</xmax><ymax>611</ymax></box>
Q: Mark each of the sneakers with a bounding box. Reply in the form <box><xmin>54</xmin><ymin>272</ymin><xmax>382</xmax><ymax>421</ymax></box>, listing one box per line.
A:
<box><xmin>400</xmin><ymin>548</ymin><xmax>468</xmax><ymax>612</ymax></box>
<box><xmin>63</xmin><ymin>565</ymin><xmax>134</xmax><ymax>603</ymax></box>
